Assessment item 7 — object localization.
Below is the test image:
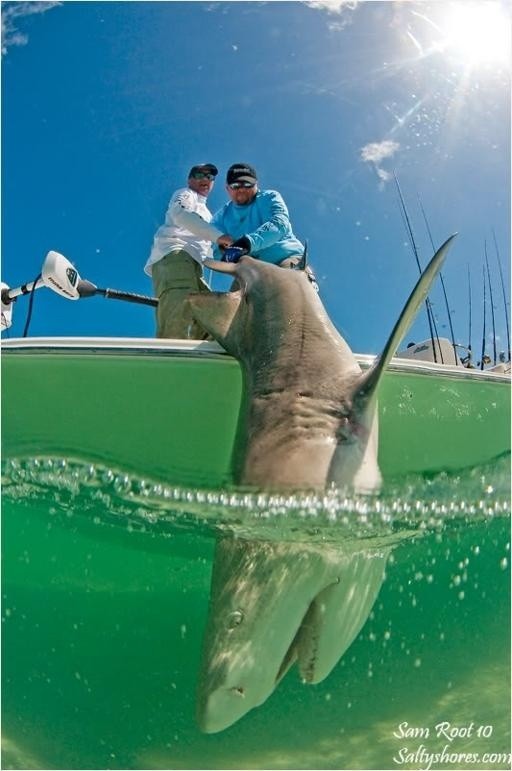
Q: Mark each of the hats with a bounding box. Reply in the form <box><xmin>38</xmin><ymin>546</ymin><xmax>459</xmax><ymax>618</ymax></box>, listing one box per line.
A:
<box><xmin>227</xmin><ymin>163</ymin><xmax>257</xmax><ymax>185</ymax></box>
<box><xmin>190</xmin><ymin>163</ymin><xmax>218</xmax><ymax>176</ymax></box>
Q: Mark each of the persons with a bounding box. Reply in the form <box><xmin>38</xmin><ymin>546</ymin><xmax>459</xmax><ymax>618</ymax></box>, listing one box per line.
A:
<box><xmin>144</xmin><ymin>162</ymin><xmax>235</xmax><ymax>338</ymax></box>
<box><xmin>209</xmin><ymin>161</ymin><xmax>322</xmax><ymax>292</ymax></box>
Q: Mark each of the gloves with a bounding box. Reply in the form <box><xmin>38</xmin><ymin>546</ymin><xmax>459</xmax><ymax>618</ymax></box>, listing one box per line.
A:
<box><xmin>221</xmin><ymin>235</ymin><xmax>250</xmax><ymax>263</ymax></box>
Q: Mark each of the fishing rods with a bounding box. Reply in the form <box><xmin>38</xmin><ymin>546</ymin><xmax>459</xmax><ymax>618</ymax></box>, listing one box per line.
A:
<box><xmin>393</xmin><ymin>169</ymin><xmax>510</xmax><ymax>370</ymax></box>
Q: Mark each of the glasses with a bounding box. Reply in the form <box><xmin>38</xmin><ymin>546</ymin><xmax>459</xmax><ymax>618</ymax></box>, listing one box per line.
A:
<box><xmin>229</xmin><ymin>182</ymin><xmax>254</xmax><ymax>189</ymax></box>
<box><xmin>190</xmin><ymin>172</ymin><xmax>215</xmax><ymax>181</ymax></box>
